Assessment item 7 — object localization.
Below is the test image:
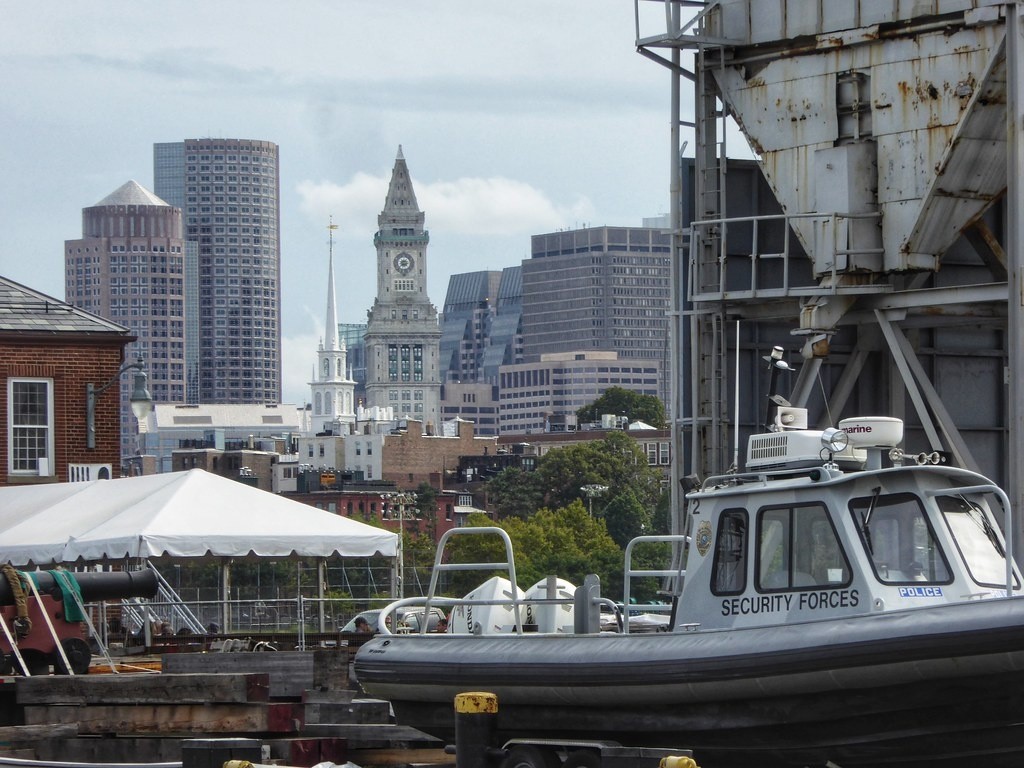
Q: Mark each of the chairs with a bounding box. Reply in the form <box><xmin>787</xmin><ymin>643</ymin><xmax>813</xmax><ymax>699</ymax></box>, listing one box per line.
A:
<box><xmin>768</xmin><ymin>570</ymin><xmax>817</xmax><ymax>589</ymax></box>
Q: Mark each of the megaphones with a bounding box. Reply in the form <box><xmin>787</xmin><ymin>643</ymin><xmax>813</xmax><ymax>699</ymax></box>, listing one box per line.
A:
<box><xmin>775</xmin><ymin>407</ymin><xmax>808</xmax><ymax>429</ymax></box>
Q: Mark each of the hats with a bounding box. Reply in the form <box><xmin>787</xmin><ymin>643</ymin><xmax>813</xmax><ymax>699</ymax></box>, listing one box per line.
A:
<box><xmin>210</xmin><ymin>622</ymin><xmax>219</xmax><ymax>632</ymax></box>
<box><xmin>355</xmin><ymin>617</ymin><xmax>371</xmax><ymax>625</ymax></box>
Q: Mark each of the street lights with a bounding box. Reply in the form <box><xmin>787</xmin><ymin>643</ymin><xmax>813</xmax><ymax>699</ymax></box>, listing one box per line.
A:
<box><xmin>380</xmin><ymin>492</ymin><xmax>420</xmax><ymax>600</ymax></box>
<box><xmin>579</xmin><ymin>483</ymin><xmax>609</xmax><ymax>520</ymax></box>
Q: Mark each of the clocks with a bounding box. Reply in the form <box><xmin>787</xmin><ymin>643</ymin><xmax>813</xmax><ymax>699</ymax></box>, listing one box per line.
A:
<box><xmin>393</xmin><ymin>252</ymin><xmax>414</xmax><ymax>274</ymax></box>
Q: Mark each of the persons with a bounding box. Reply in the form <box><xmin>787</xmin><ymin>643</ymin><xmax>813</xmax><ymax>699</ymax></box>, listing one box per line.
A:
<box><xmin>139</xmin><ymin>621</ymin><xmax>192</xmax><ymax>635</ymax></box>
<box><xmin>437</xmin><ymin>619</ymin><xmax>447</xmax><ymax>632</ymax></box>
<box><xmin>354</xmin><ymin>617</ymin><xmax>372</xmax><ymax>632</ymax></box>
<box><xmin>101</xmin><ymin>618</ymin><xmax>132</xmax><ymax>635</ymax></box>
<box><xmin>207</xmin><ymin>622</ymin><xmax>220</xmax><ymax>634</ymax></box>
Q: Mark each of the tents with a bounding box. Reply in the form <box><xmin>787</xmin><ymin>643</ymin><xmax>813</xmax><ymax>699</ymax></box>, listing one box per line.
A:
<box><xmin>0</xmin><ymin>468</ymin><xmax>404</xmax><ymax>654</ymax></box>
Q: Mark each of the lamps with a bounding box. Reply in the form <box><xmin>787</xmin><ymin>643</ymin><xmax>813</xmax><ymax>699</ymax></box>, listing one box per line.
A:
<box><xmin>678</xmin><ymin>472</ymin><xmax>702</xmax><ymax>493</ymax></box>
<box><xmin>765</xmin><ymin>344</ymin><xmax>795</xmax><ymax>371</ymax></box>
<box><xmin>821</xmin><ymin>427</ymin><xmax>849</xmax><ymax>471</ymax></box>
<box><xmin>85</xmin><ymin>354</ymin><xmax>155</xmax><ymax>448</ymax></box>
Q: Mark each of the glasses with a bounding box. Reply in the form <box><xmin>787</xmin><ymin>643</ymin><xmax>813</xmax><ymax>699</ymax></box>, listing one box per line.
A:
<box><xmin>386</xmin><ymin>622</ymin><xmax>391</xmax><ymax>624</ymax></box>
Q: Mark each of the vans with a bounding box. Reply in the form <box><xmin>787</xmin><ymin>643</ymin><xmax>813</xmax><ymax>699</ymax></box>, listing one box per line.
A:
<box><xmin>325</xmin><ymin>606</ymin><xmax>445</xmax><ymax>648</ymax></box>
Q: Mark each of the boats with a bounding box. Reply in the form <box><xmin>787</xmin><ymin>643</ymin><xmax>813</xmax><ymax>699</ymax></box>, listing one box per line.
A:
<box><xmin>352</xmin><ymin>320</ymin><xmax>1024</xmax><ymax>768</ymax></box>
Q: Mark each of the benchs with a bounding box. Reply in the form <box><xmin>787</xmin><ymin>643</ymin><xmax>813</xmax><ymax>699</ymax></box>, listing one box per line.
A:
<box><xmin>874</xmin><ymin>569</ymin><xmax>927</xmax><ymax>583</ymax></box>
